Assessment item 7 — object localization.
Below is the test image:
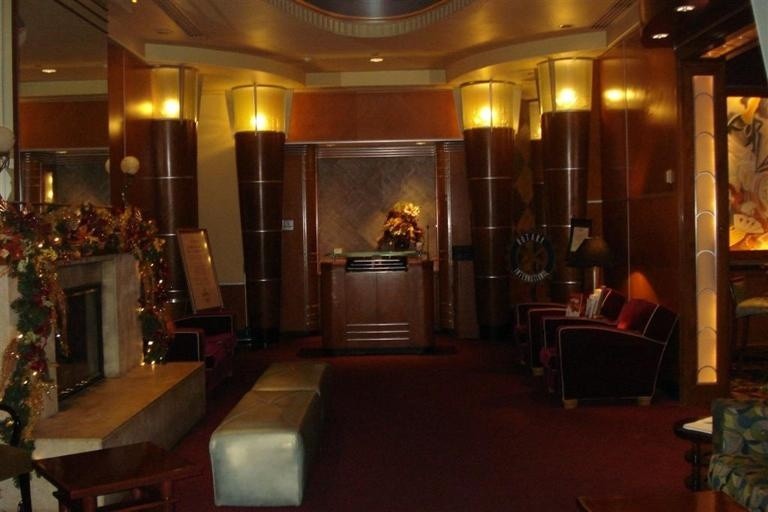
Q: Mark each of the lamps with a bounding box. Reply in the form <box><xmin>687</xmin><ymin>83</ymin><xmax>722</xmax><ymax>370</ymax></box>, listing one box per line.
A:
<box><xmin>566</xmin><ymin>238</ymin><xmax>611</xmax><ymax>292</ymax></box>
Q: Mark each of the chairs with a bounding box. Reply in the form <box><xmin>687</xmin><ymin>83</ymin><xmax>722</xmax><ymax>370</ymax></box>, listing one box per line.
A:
<box><xmin>707</xmin><ymin>395</ymin><xmax>768</xmax><ymax>512</ymax></box>
<box><xmin>515</xmin><ymin>285</ymin><xmax>680</xmax><ymax>410</ymax></box>
<box><xmin>727</xmin><ymin>280</ymin><xmax>768</xmax><ymax>376</ymax></box>
<box><xmin>0</xmin><ymin>403</ymin><xmax>33</xmax><ymax>512</ymax></box>
<box><xmin>162</xmin><ymin>311</ymin><xmax>238</xmax><ymax>414</ymax></box>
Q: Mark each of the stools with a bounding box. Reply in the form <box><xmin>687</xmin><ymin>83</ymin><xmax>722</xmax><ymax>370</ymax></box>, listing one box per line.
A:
<box><xmin>209</xmin><ymin>359</ymin><xmax>333</xmax><ymax>506</ymax></box>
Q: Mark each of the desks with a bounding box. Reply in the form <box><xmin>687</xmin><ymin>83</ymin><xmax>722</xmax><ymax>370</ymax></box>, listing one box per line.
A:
<box><xmin>31</xmin><ymin>442</ymin><xmax>202</xmax><ymax>512</ymax></box>
<box><xmin>319</xmin><ymin>250</ymin><xmax>436</xmax><ymax>355</ymax></box>
<box><xmin>673</xmin><ymin>415</ymin><xmax>713</xmax><ymax>492</ymax></box>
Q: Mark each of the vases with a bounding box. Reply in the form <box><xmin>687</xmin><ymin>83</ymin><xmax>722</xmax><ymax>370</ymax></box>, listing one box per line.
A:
<box><xmin>395</xmin><ymin>237</ymin><xmax>410</xmax><ymax>251</ymax></box>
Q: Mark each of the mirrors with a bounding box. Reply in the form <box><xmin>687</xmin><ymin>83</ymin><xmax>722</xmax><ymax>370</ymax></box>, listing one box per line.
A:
<box><xmin>11</xmin><ymin>1</ymin><xmax>111</xmax><ymax>218</ymax></box>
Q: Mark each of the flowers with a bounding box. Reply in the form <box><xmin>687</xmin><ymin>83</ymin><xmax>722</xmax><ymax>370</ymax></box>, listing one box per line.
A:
<box><xmin>383</xmin><ymin>201</ymin><xmax>425</xmax><ymax>242</ymax></box>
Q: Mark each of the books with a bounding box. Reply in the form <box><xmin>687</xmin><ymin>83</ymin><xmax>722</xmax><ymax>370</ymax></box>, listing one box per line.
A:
<box><xmin>564</xmin><ymin>286</ymin><xmax>603</xmax><ymax>318</ymax></box>
